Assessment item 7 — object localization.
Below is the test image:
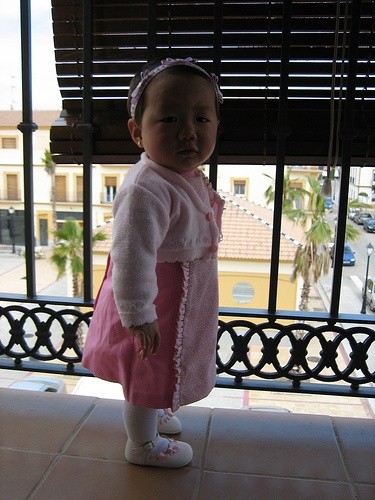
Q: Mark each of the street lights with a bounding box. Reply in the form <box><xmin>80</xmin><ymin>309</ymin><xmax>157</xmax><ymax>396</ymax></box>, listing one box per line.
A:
<box><xmin>330</xmin><ymin>216</ymin><xmax>337</xmax><ymax>268</ymax></box>
<box><xmin>8</xmin><ymin>206</ymin><xmax>16</xmax><ymax>254</ymax></box>
<box><xmin>360</xmin><ymin>242</ymin><xmax>374</xmax><ymax>314</ymax></box>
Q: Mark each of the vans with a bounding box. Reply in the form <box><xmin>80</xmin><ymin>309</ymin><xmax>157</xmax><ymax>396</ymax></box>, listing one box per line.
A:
<box><xmin>348</xmin><ymin>208</ymin><xmax>360</xmax><ymax>220</ymax></box>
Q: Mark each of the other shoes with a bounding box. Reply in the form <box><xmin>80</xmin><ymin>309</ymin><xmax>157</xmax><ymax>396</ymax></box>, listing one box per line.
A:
<box><xmin>124</xmin><ymin>434</ymin><xmax>193</xmax><ymax>468</ymax></box>
<box><xmin>156</xmin><ymin>410</ymin><xmax>181</xmax><ymax>434</ymax></box>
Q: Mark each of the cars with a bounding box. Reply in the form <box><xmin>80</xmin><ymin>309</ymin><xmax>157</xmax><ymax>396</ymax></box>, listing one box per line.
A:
<box><xmin>330</xmin><ymin>243</ymin><xmax>356</xmax><ymax>266</ymax></box>
<box><xmin>353</xmin><ymin>212</ymin><xmax>372</xmax><ymax>225</ymax></box>
<box><xmin>363</xmin><ymin>218</ymin><xmax>375</xmax><ymax>233</ymax></box>
<box><xmin>325</xmin><ymin>198</ymin><xmax>333</xmax><ymax>209</ymax></box>
<box><xmin>362</xmin><ymin>278</ymin><xmax>375</xmax><ymax>312</ymax></box>
<box><xmin>328</xmin><ymin>234</ymin><xmax>335</xmax><ymax>253</ymax></box>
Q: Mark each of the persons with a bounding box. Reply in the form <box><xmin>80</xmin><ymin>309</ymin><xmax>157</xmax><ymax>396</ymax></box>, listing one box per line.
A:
<box><xmin>80</xmin><ymin>58</ymin><xmax>227</xmax><ymax>468</ymax></box>
